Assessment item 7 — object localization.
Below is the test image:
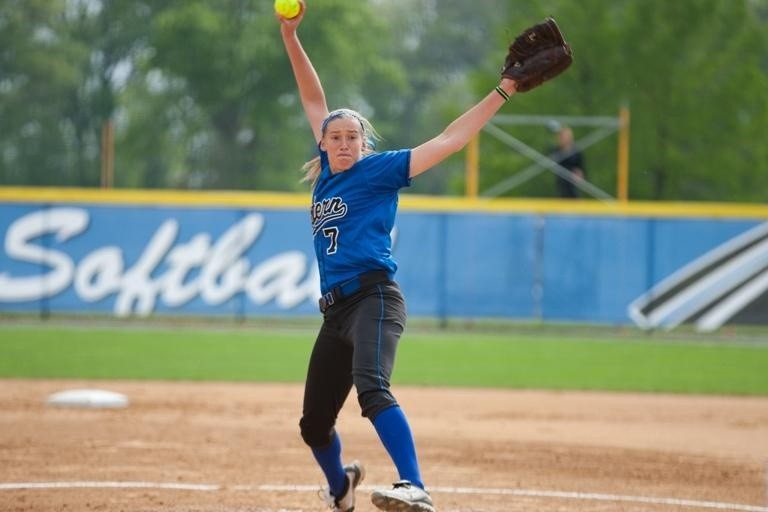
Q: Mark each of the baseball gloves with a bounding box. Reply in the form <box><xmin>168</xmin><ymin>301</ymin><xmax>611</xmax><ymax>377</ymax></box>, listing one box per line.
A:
<box><xmin>501</xmin><ymin>16</ymin><xmax>572</xmax><ymax>92</ymax></box>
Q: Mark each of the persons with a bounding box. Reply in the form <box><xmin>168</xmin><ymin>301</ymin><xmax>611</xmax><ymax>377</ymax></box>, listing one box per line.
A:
<box><xmin>545</xmin><ymin>120</ymin><xmax>588</xmax><ymax>200</ymax></box>
<box><xmin>274</xmin><ymin>0</ymin><xmax>577</xmax><ymax>511</ymax></box>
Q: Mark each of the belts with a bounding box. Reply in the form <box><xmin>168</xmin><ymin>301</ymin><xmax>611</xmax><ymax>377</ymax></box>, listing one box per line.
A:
<box><xmin>318</xmin><ymin>268</ymin><xmax>394</xmax><ymax>312</ymax></box>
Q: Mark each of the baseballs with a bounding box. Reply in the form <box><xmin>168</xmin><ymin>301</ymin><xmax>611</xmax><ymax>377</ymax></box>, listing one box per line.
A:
<box><xmin>275</xmin><ymin>0</ymin><xmax>300</xmax><ymax>20</ymax></box>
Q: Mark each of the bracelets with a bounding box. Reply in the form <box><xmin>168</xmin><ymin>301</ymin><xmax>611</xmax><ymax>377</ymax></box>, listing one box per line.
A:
<box><xmin>493</xmin><ymin>85</ymin><xmax>513</xmax><ymax>103</ymax></box>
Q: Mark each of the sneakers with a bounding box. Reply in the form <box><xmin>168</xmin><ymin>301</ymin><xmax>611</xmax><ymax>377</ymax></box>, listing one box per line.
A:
<box><xmin>372</xmin><ymin>479</ymin><xmax>434</xmax><ymax>512</ymax></box>
<box><xmin>333</xmin><ymin>461</ymin><xmax>365</xmax><ymax>512</ymax></box>
